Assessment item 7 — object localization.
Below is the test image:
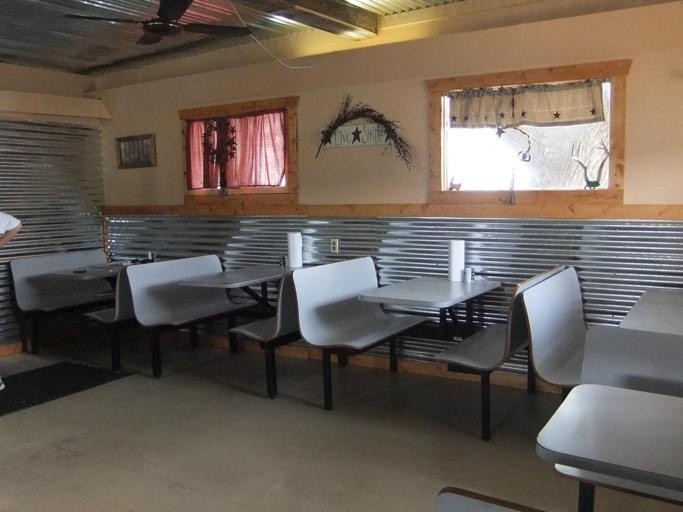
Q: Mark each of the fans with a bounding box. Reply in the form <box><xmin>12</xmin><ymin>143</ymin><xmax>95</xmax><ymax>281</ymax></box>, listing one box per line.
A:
<box><xmin>62</xmin><ymin>0</ymin><xmax>251</xmax><ymax>46</ymax></box>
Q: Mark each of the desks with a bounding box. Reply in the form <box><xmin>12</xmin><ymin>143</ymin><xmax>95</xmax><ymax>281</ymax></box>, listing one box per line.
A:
<box><xmin>359</xmin><ymin>276</ymin><xmax>500</xmax><ymax>308</ymax></box>
<box><xmin>617</xmin><ymin>286</ymin><xmax>683</xmax><ymax>331</ymax></box>
<box><xmin>179</xmin><ymin>272</ymin><xmax>283</xmax><ymax>287</ymax></box>
<box><xmin>534</xmin><ymin>384</ymin><xmax>682</xmax><ymax>512</ymax></box>
<box><xmin>47</xmin><ymin>265</ymin><xmax>121</xmax><ymax>285</ymax></box>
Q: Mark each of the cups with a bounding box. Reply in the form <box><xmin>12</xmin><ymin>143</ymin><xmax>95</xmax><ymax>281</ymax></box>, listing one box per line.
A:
<box><xmin>147</xmin><ymin>252</ymin><xmax>155</xmax><ymax>261</ymax></box>
<box><xmin>280</xmin><ymin>256</ymin><xmax>288</xmax><ymax>268</ymax></box>
<box><xmin>465</xmin><ymin>268</ymin><xmax>475</xmax><ymax>284</ymax></box>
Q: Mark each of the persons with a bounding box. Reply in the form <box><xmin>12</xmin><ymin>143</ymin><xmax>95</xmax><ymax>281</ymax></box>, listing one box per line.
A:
<box><xmin>0</xmin><ymin>209</ymin><xmax>22</xmax><ymax>392</ymax></box>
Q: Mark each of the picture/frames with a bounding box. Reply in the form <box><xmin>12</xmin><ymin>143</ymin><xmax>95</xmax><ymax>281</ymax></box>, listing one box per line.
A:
<box><xmin>115</xmin><ymin>133</ymin><xmax>156</xmax><ymax>169</ymax></box>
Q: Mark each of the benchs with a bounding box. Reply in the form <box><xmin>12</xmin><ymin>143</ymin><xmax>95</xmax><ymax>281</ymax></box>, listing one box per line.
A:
<box><xmin>229</xmin><ymin>271</ymin><xmax>297</xmax><ymax>398</ymax></box>
<box><xmin>127</xmin><ymin>254</ymin><xmax>258</xmax><ymax>381</ymax></box>
<box><xmin>522</xmin><ymin>266</ymin><xmax>683</xmax><ymax>399</ymax></box>
<box><xmin>10</xmin><ymin>250</ymin><xmax>107</xmax><ymax>353</ymax></box>
<box><xmin>555</xmin><ymin>327</ymin><xmax>682</xmax><ymax>511</ymax></box>
<box><xmin>435</xmin><ymin>267</ymin><xmax>564</xmax><ymax>438</ymax></box>
<box><xmin>82</xmin><ymin>267</ymin><xmax>131</xmax><ymax>363</ymax></box>
<box><xmin>292</xmin><ymin>256</ymin><xmax>426</xmax><ymax>411</ymax></box>
